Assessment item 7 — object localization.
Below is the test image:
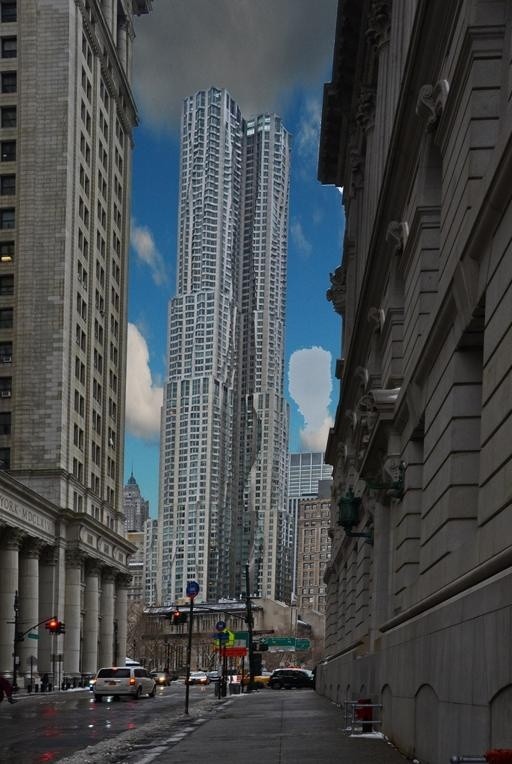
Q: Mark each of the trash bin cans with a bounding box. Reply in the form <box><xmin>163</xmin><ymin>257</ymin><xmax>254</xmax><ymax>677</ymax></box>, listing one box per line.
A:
<box><xmin>229</xmin><ymin>684</ymin><xmax>241</xmax><ymax>694</ymax></box>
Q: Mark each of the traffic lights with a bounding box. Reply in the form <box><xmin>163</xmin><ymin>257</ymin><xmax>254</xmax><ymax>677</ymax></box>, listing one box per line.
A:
<box><xmin>58</xmin><ymin>621</ymin><xmax>66</xmax><ymax>636</ymax></box>
<box><xmin>164</xmin><ymin>612</ymin><xmax>173</xmax><ymax>621</ymax></box>
<box><xmin>173</xmin><ymin>611</ymin><xmax>181</xmax><ymax>625</ymax></box>
<box><xmin>45</xmin><ymin>621</ymin><xmax>59</xmax><ymax>630</ymax></box>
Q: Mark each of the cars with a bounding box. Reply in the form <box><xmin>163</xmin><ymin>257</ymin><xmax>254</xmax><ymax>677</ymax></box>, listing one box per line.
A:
<box><xmin>188</xmin><ymin>670</ymin><xmax>219</xmax><ymax>686</ymax></box>
<box><xmin>242</xmin><ymin>670</ymin><xmax>272</xmax><ymax>687</ymax></box>
<box><xmin>151</xmin><ymin>672</ymin><xmax>172</xmax><ymax>687</ymax></box>
<box><xmin>88</xmin><ymin>676</ymin><xmax>96</xmax><ymax>690</ymax></box>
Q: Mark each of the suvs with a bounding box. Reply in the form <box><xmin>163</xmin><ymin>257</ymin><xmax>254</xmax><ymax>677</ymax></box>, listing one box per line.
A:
<box><xmin>267</xmin><ymin>668</ymin><xmax>315</xmax><ymax>691</ymax></box>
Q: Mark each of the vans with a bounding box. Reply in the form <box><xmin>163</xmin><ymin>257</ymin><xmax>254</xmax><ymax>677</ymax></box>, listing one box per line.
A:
<box><xmin>92</xmin><ymin>666</ymin><xmax>159</xmax><ymax>700</ymax></box>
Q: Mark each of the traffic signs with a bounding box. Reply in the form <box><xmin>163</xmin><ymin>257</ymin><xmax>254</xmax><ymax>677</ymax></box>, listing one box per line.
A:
<box><xmin>259</xmin><ymin>637</ymin><xmax>296</xmax><ymax>645</ymax></box>
<box><xmin>296</xmin><ymin>638</ymin><xmax>312</xmax><ymax>652</ymax></box>
<box><xmin>27</xmin><ymin>634</ymin><xmax>40</xmax><ymax>640</ymax></box>
<box><xmin>231</xmin><ymin>632</ymin><xmax>250</xmax><ymax>647</ymax></box>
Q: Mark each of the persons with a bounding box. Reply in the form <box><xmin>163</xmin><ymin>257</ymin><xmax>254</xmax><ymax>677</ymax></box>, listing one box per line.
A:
<box><xmin>0</xmin><ymin>674</ymin><xmax>18</xmax><ymax>705</ymax></box>
<box><xmin>34</xmin><ymin>671</ymin><xmax>48</xmax><ymax>690</ymax></box>
